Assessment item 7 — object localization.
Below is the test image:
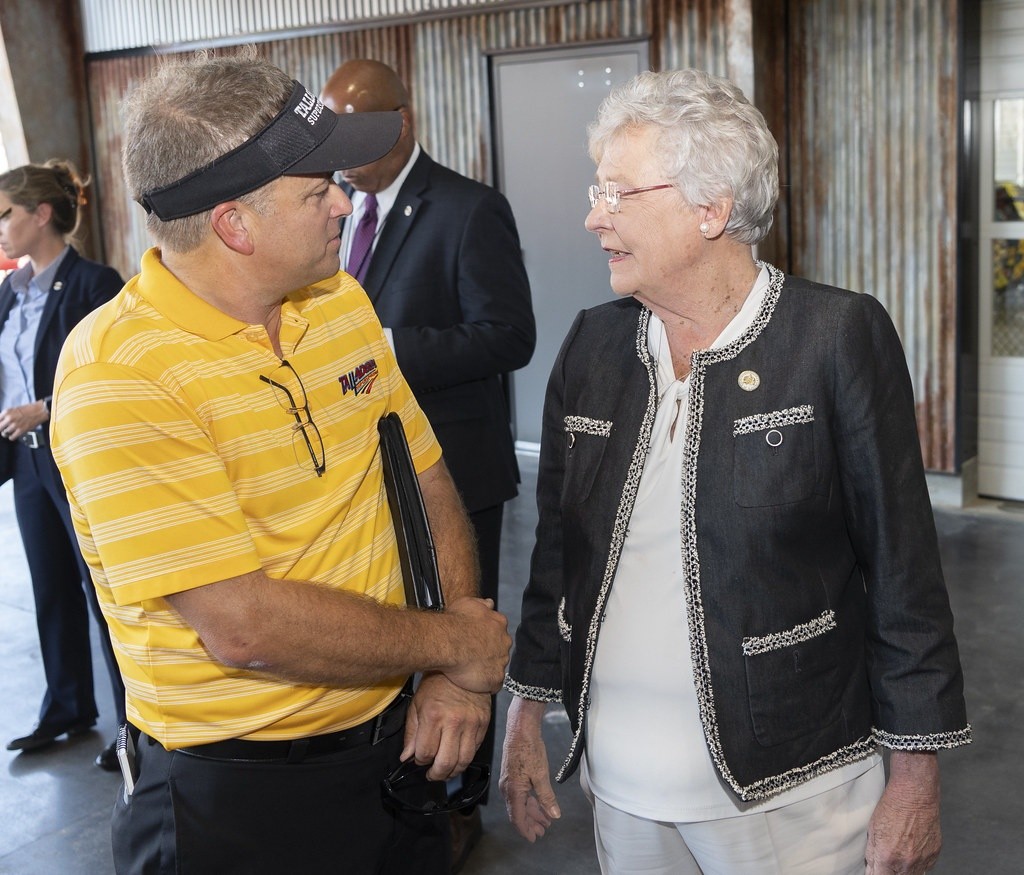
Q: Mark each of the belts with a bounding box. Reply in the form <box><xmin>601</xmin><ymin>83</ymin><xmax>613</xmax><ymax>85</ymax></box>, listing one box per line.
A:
<box><xmin>173</xmin><ymin>691</ymin><xmax>412</xmax><ymax>763</ymax></box>
<box><xmin>15</xmin><ymin>430</ymin><xmax>48</xmax><ymax>449</ymax></box>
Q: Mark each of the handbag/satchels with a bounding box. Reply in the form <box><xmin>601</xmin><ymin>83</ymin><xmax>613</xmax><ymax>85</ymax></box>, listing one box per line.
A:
<box><xmin>376</xmin><ymin>411</ymin><xmax>446</xmax><ymax>613</ymax></box>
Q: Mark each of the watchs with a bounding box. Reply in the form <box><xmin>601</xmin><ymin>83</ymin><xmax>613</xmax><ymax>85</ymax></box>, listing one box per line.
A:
<box><xmin>42</xmin><ymin>396</ymin><xmax>52</xmax><ymax>412</ymax></box>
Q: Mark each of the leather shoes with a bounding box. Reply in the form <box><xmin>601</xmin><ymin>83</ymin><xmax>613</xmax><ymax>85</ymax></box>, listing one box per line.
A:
<box><xmin>93</xmin><ymin>726</ymin><xmax>140</xmax><ymax>771</ymax></box>
<box><xmin>6</xmin><ymin>711</ymin><xmax>96</xmax><ymax>751</ymax></box>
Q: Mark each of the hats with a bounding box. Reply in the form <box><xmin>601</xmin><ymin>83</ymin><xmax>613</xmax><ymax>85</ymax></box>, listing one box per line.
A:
<box><xmin>134</xmin><ymin>79</ymin><xmax>404</xmax><ymax>222</ymax></box>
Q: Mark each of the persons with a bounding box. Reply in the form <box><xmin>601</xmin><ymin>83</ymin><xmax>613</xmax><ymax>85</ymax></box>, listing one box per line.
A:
<box><xmin>318</xmin><ymin>57</ymin><xmax>540</xmax><ymax>802</ymax></box>
<box><xmin>497</xmin><ymin>69</ymin><xmax>971</xmax><ymax>874</ymax></box>
<box><xmin>50</xmin><ymin>56</ymin><xmax>509</xmax><ymax>874</ymax></box>
<box><xmin>0</xmin><ymin>160</ymin><xmax>127</xmax><ymax>769</ymax></box>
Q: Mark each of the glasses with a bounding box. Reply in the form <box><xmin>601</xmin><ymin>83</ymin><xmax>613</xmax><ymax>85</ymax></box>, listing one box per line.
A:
<box><xmin>379</xmin><ymin>755</ymin><xmax>492</xmax><ymax>820</ymax></box>
<box><xmin>259</xmin><ymin>358</ymin><xmax>326</xmax><ymax>479</ymax></box>
<box><xmin>586</xmin><ymin>180</ymin><xmax>680</xmax><ymax>215</ymax></box>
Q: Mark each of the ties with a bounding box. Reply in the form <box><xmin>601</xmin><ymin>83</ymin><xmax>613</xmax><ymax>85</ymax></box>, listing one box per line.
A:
<box><xmin>346</xmin><ymin>191</ymin><xmax>378</xmax><ymax>287</ymax></box>
